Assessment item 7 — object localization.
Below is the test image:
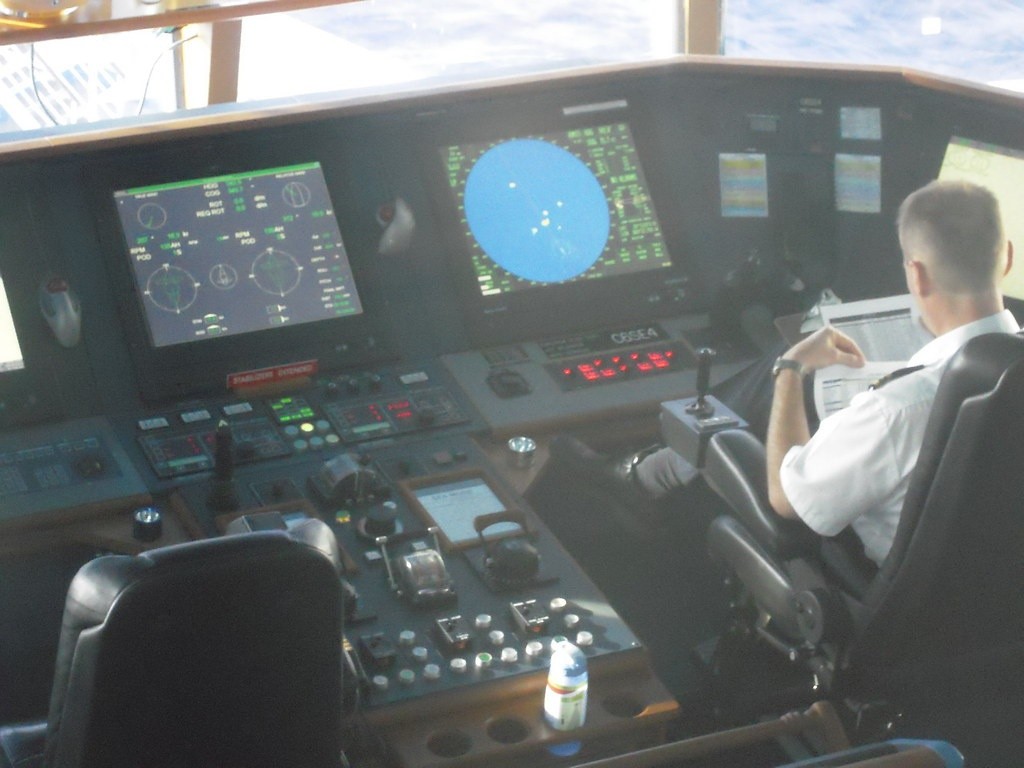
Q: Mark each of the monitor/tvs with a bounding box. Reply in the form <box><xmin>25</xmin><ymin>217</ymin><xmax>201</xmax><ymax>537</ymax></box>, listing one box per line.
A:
<box><xmin>413</xmin><ymin>84</ymin><xmax>709</xmax><ymax>353</ymax></box>
<box><xmin>937</xmin><ymin>135</ymin><xmax>1024</xmax><ymax>302</ymax></box>
<box><xmin>87</xmin><ymin>147</ymin><xmax>402</xmax><ymax>406</ymax></box>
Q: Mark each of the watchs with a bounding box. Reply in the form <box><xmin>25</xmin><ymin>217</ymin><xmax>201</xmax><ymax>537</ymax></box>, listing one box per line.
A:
<box><xmin>772</xmin><ymin>356</ymin><xmax>809</xmax><ymax>381</ymax></box>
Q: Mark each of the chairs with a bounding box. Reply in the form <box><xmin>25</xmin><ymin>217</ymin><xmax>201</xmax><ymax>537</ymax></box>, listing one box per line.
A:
<box><xmin>656</xmin><ymin>329</ymin><xmax>1024</xmax><ymax>690</ymax></box>
<box><xmin>0</xmin><ymin>518</ymin><xmax>359</xmax><ymax>768</ymax></box>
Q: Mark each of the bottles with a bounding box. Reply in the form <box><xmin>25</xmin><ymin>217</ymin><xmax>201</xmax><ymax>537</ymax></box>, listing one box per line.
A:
<box><xmin>542</xmin><ymin>639</ymin><xmax>588</xmax><ymax>758</ymax></box>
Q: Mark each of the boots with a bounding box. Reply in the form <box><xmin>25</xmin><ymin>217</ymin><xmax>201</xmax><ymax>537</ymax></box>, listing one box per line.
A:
<box><xmin>552</xmin><ymin>435</ymin><xmax>670</xmax><ymax>539</ymax></box>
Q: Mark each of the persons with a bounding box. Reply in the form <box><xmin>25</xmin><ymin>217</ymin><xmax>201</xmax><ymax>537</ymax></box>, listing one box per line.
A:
<box><xmin>548</xmin><ymin>177</ymin><xmax>1024</xmax><ymax>614</ymax></box>
